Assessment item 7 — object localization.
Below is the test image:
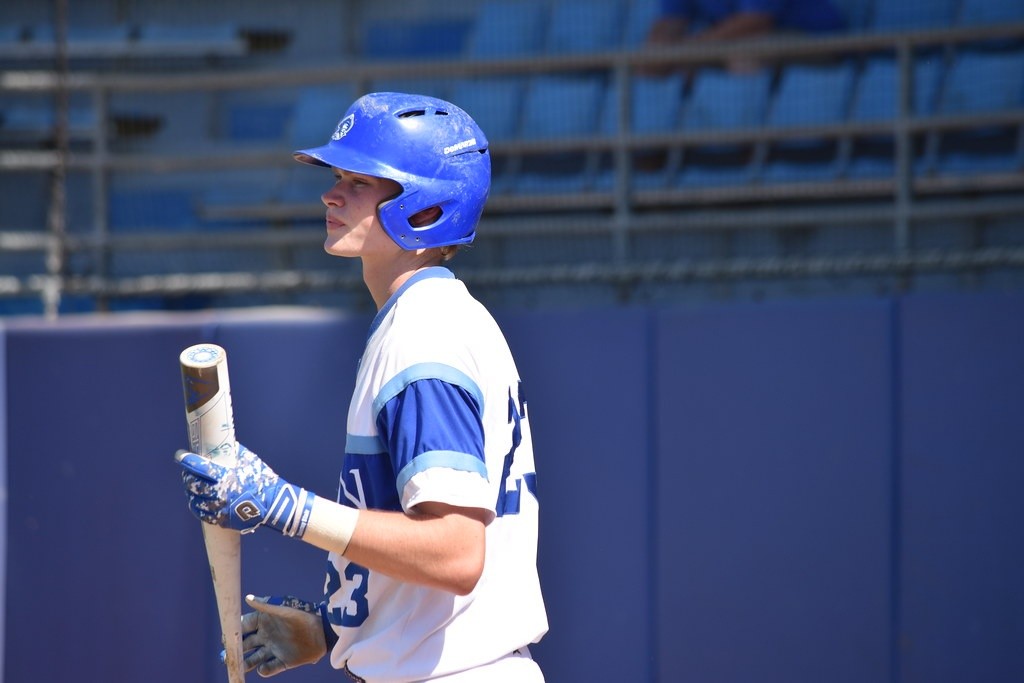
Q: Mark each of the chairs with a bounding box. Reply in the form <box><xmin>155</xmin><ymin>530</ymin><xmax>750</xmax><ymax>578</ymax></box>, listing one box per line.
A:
<box><xmin>3</xmin><ymin>0</ymin><xmax>1023</xmax><ymax>287</ymax></box>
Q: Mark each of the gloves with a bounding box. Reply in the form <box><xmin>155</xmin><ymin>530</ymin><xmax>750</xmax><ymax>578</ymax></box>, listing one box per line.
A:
<box><xmin>219</xmin><ymin>593</ymin><xmax>337</xmax><ymax>677</ymax></box>
<box><xmin>175</xmin><ymin>439</ymin><xmax>359</xmax><ymax>557</ymax></box>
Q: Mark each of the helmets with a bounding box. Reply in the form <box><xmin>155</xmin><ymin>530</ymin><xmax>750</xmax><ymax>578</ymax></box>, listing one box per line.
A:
<box><xmin>292</xmin><ymin>91</ymin><xmax>492</xmax><ymax>250</ymax></box>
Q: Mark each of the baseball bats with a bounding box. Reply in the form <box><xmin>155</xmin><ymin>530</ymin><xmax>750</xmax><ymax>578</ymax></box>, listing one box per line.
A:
<box><xmin>178</xmin><ymin>342</ymin><xmax>246</xmax><ymax>683</ymax></box>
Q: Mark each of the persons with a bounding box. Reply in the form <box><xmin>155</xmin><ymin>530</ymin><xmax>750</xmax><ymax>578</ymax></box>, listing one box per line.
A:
<box><xmin>173</xmin><ymin>94</ymin><xmax>550</xmax><ymax>683</ymax></box>
